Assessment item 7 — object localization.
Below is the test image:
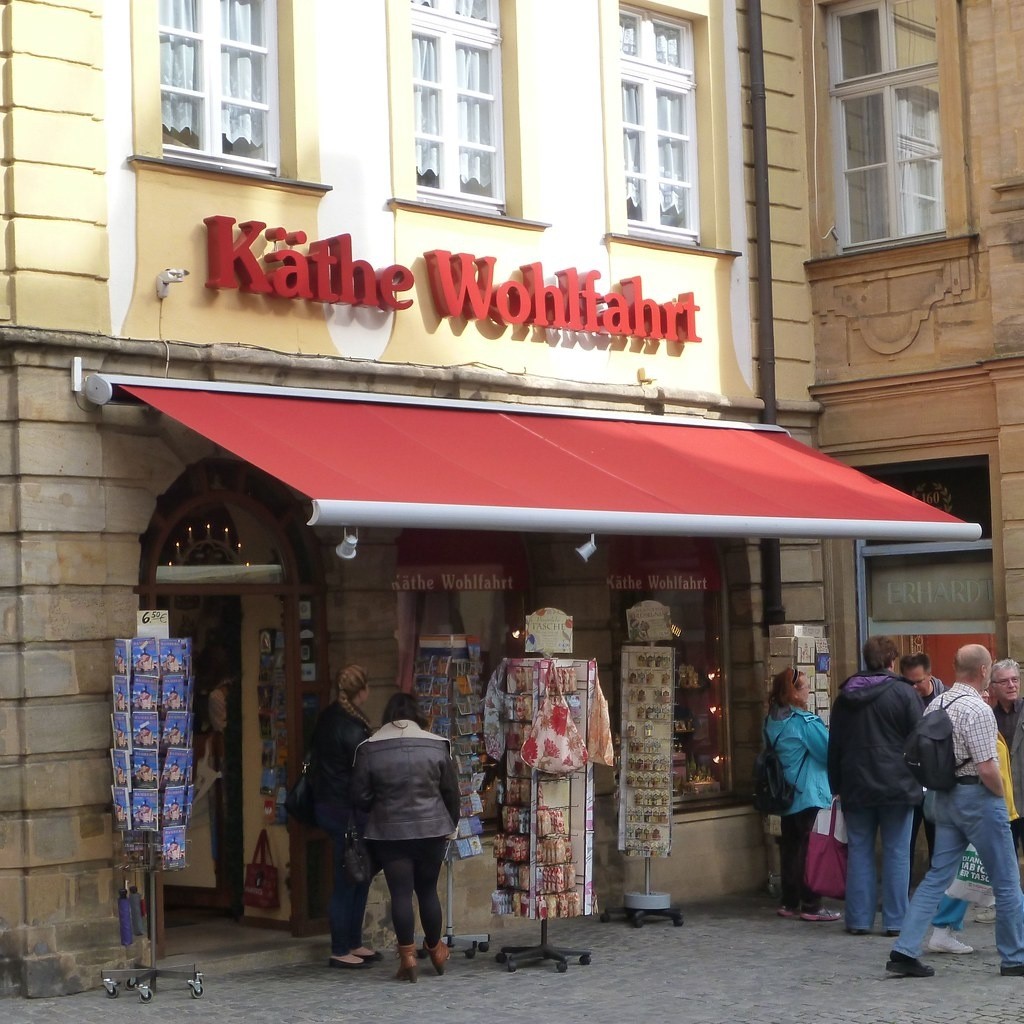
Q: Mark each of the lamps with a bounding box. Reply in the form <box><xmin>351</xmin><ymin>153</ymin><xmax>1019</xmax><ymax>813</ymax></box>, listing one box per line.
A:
<box><xmin>335</xmin><ymin>526</ymin><xmax>358</xmax><ymax>560</ymax></box>
<box><xmin>575</xmin><ymin>532</ymin><xmax>597</xmax><ymax>563</ymax></box>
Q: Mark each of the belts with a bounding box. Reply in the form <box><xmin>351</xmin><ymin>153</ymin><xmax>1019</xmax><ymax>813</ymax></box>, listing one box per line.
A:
<box><xmin>957</xmin><ymin>774</ymin><xmax>984</xmax><ymax>785</ymax></box>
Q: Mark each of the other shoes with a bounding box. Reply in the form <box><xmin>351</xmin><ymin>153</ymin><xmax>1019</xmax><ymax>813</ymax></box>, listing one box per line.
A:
<box><xmin>976</xmin><ymin>906</ymin><xmax>998</xmax><ymax>924</ymax></box>
<box><xmin>330</xmin><ymin>956</ymin><xmax>373</xmax><ymax>967</ymax></box>
<box><xmin>800</xmin><ymin>907</ymin><xmax>843</xmax><ymax>920</ymax></box>
<box><xmin>353</xmin><ymin>951</ymin><xmax>383</xmax><ymax>961</ymax></box>
<box><xmin>777</xmin><ymin>903</ymin><xmax>801</xmax><ymax>916</ymax></box>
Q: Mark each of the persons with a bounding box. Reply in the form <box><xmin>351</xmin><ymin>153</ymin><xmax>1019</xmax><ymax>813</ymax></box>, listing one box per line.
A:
<box><xmin>976</xmin><ymin>660</ymin><xmax>1024</xmax><ymax>923</ymax></box>
<box><xmin>350</xmin><ymin>692</ymin><xmax>461</xmax><ymax>982</ymax></box>
<box><xmin>761</xmin><ymin>669</ymin><xmax>840</xmax><ymax>921</ymax></box>
<box><xmin>827</xmin><ymin>636</ymin><xmax>921</xmax><ymax>935</ymax></box>
<box><xmin>312</xmin><ymin>664</ymin><xmax>385</xmax><ymax>969</ymax></box>
<box><xmin>887</xmin><ymin>644</ymin><xmax>1024</xmax><ymax>977</ymax></box>
<box><xmin>900</xmin><ymin>653</ymin><xmax>954</xmax><ymax>891</ymax></box>
<box><xmin>928</xmin><ymin>680</ymin><xmax>989</xmax><ymax>954</ymax></box>
<box><xmin>207</xmin><ymin>629</ymin><xmax>243</xmax><ymax>918</ymax></box>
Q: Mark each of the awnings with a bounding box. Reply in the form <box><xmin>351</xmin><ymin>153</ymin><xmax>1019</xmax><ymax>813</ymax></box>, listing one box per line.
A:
<box><xmin>86</xmin><ymin>373</ymin><xmax>983</xmax><ymax>542</ymax></box>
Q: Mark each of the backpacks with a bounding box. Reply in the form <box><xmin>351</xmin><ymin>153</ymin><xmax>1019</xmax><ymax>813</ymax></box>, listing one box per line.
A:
<box><xmin>903</xmin><ymin>693</ymin><xmax>975</xmax><ymax>790</ymax></box>
<box><xmin>752</xmin><ymin>713</ymin><xmax>810</xmax><ymax>813</ymax></box>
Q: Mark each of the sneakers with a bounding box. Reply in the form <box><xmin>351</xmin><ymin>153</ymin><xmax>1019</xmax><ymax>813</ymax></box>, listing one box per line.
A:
<box><xmin>927</xmin><ymin>936</ymin><xmax>973</xmax><ymax>953</ymax></box>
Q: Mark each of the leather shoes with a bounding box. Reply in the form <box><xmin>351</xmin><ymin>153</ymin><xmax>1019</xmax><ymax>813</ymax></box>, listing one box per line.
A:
<box><xmin>1001</xmin><ymin>963</ymin><xmax>1024</xmax><ymax>976</ymax></box>
<box><xmin>885</xmin><ymin>951</ymin><xmax>934</xmax><ymax>975</ymax></box>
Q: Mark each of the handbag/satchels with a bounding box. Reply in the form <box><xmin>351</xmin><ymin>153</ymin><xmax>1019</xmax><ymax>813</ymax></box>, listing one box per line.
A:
<box><xmin>341</xmin><ymin>837</ymin><xmax>373</xmax><ymax>883</ymax></box>
<box><xmin>803</xmin><ymin>798</ymin><xmax>847</xmax><ymax>900</ymax></box>
<box><xmin>944</xmin><ymin>842</ymin><xmax>996</xmax><ymax>906</ymax></box>
<box><xmin>519</xmin><ymin>660</ymin><xmax>587</xmax><ymax>772</ymax></box>
<box><xmin>283</xmin><ymin>764</ymin><xmax>312</xmax><ymax>828</ymax></box>
<box><xmin>241</xmin><ymin>829</ymin><xmax>281</xmax><ymax>908</ymax></box>
<box><xmin>589</xmin><ymin>660</ymin><xmax>615</xmax><ymax>767</ymax></box>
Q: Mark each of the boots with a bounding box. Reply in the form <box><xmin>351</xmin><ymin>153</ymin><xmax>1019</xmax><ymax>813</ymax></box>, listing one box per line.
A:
<box><xmin>425</xmin><ymin>939</ymin><xmax>449</xmax><ymax>975</ymax></box>
<box><xmin>393</xmin><ymin>943</ymin><xmax>418</xmax><ymax>984</ymax></box>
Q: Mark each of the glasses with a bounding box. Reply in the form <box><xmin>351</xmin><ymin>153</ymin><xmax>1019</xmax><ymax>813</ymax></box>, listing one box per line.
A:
<box><xmin>993</xmin><ymin>677</ymin><xmax>1020</xmax><ymax>685</ymax></box>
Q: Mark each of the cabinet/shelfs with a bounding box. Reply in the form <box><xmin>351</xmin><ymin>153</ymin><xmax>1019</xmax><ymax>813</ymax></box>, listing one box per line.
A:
<box><xmin>597</xmin><ymin>645</ymin><xmax>684</xmax><ymax>928</ymax></box>
<box><xmin>492</xmin><ymin>658</ymin><xmax>599</xmax><ymax>971</ymax></box>
<box><xmin>406</xmin><ymin>653</ymin><xmax>491</xmax><ymax>961</ymax></box>
<box><xmin>764</xmin><ymin>621</ymin><xmax>830</xmax><ymax>901</ymax></box>
<box><xmin>99</xmin><ymin>637</ymin><xmax>206</xmax><ymax>1005</ymax></box>
